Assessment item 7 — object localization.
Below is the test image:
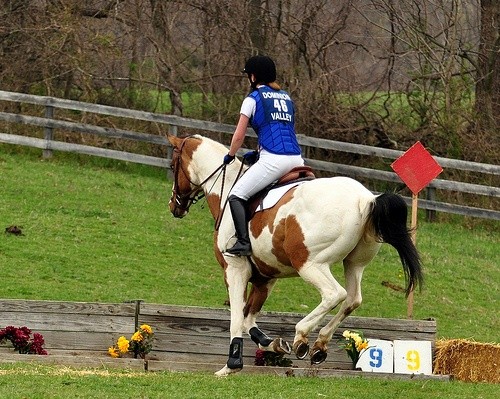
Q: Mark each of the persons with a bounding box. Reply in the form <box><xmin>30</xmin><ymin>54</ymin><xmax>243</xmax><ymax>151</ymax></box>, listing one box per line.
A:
<box><xmin>223</xmin><ymin>55</ymin><xmax>304</xmax><ymax>256</ymax></box>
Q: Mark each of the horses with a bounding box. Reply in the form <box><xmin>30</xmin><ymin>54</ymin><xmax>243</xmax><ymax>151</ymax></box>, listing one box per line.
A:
<box><xmin>166</xmin><ymin>134</ymin><xmax>425</xmax><ymax>375</ymax></box>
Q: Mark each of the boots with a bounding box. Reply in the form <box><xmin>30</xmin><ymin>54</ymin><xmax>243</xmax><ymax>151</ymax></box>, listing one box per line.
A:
<box><xmin>226</xmin><ymin>195</ymin><xmax>253</xmax><ymax>256</ymax></box>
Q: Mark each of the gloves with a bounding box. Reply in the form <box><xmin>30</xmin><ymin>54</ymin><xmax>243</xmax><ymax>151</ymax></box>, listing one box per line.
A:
<box><xmin>223</xmin><ymin>152</ymin><xmax>235</xmax><ymax>165</ymax></box>
<box><xmin>242</xmin><ymin>150</ymin><xmax>258</xmax><ymax>165</ymax></box>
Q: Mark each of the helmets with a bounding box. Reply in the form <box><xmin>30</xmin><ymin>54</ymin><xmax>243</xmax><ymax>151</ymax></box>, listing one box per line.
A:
<box><xmin>240</xmin><ymin>56</ymin><xmax>276</xmax><ymax>84</ymax></box>
